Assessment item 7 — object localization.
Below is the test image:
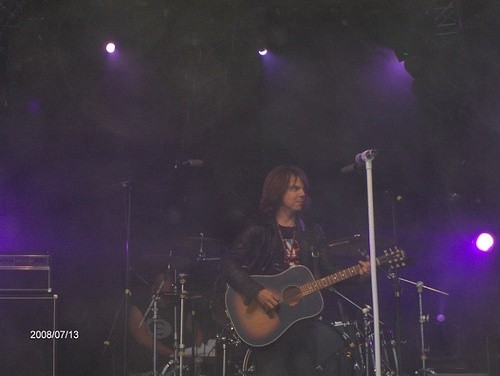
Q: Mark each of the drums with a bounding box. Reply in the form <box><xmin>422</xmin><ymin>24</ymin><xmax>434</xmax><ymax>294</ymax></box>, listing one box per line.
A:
<box><xmin>225</xmin><ymin>315</ymin><xmax>366</xmax><ymax>376</ymax></box>
<box><xmin>329</xmin><ymin>319</ymin><xmax>365</xmax><ymax>345</ymax></box>
<box><xmin>213</xmin><ymin>332</ymin><xmax>258</xmax><ymax>376</ymax></box>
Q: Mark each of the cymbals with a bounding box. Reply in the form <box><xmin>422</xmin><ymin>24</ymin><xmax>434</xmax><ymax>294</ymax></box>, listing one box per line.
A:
<box><xmin>144</xmin><ymin>252</ymin><xmax>183</xmax><ymax>260</ymax></box>
<box><xmin>165</xmin><ymin>288</ymin><xmax>202</xmax><ymax>300</ymax></box>
<box><xmin>186</xmin><ymin>235</ymin><xmax>223</xmax><ymax>245</ymax></box>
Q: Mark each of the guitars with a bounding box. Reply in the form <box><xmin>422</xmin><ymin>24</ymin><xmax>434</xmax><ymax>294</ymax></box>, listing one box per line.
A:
<box><xmin>224</xmin><ymin>244</ymin><xmax>412</xmax><ymax>349</ymax></box>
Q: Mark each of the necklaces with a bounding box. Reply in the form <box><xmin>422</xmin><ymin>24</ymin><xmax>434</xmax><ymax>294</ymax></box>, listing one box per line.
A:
<box><xmin>279</xmin><ymin>225</ymin><xmax>296</xmax><ymax>261</ymax></box>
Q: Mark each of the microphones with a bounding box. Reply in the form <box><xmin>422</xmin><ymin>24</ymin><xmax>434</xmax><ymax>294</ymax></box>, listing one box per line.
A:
<box><xmin>175</xmin><ymin>159</ymin><xmax>204</xmax><ymax>170</ymax></box>
<box><xmin>356</xmin><ymin>150</ymin><xmax>377</xmax><ymax>165</ymax></box>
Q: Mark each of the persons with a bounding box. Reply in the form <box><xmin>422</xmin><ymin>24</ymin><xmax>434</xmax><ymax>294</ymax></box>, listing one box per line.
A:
<box><xmin>129</xmin><ymin>166</ymin><xmax>370</xmax><ymax>376</ymax></box>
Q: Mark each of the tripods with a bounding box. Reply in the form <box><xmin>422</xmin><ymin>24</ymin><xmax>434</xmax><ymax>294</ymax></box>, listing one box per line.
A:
<box><xmin>160</xmin><ymin>270</ymin><xmax>191</xmax><ymax>376</ymax></box>
<box><xmin>398</xmin><ymin>278</ymin><xmax>450</xmax><ymax>376</ymax></box>
